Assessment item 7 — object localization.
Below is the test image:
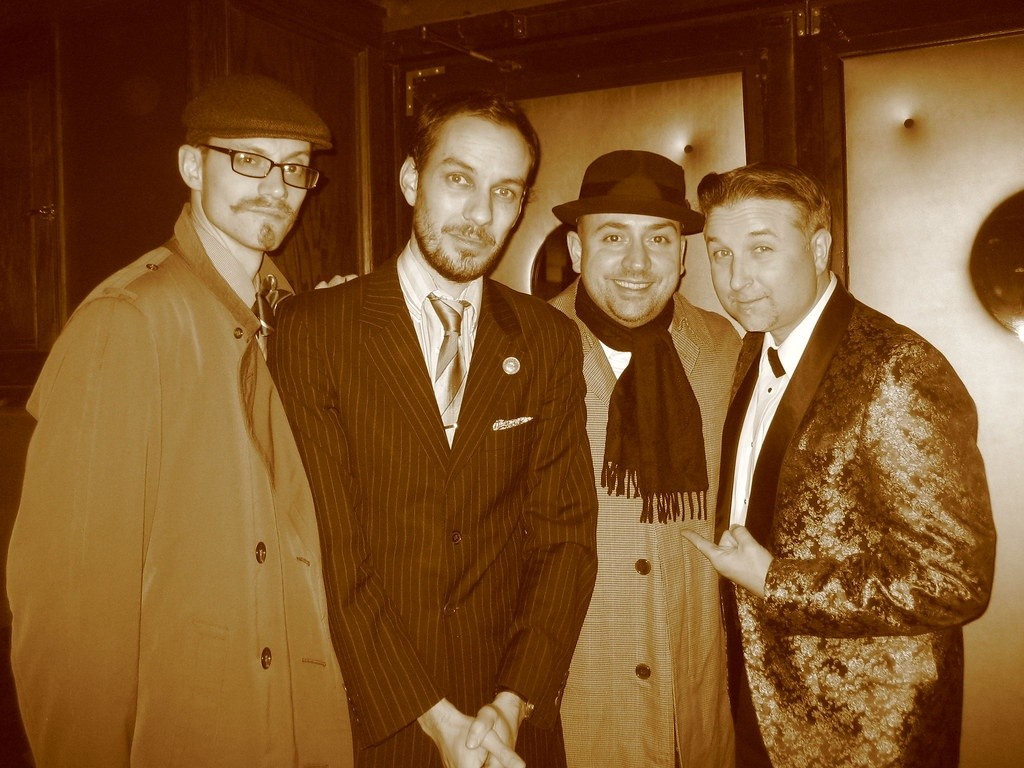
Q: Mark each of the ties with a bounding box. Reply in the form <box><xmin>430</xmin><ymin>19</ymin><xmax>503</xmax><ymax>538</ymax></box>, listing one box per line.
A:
<box><xmin>427</xmin><ymin>292</ymin><xmax>471</xmax><ymax>449</ymax></box>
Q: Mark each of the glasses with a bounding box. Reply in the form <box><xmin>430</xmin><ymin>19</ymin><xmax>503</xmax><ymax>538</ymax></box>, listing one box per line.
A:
<box><xmin>195</xmin><ymin>144</ymin><xmax>319</xmax><ymax>189</ymax></box>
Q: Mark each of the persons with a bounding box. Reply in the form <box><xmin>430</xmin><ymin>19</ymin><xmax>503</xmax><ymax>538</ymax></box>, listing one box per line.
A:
<box><xmin>5</xmin><ymin>75</ymin><xmax>357</xmax><ymax>768</ymax></box>
<box><xmin>535</xmin><ymin>150</ymin><xmax>743</xmax><ymax>768</ymax></box>
<box><xmin>680</xmin><ymin>161</ymin><xmax>998</xmax><ymax>768</ymax></box>
<box><xmin>264</xmin><ymin>86</ymin><xmax>599</xmax><ymax>767</ymax></box>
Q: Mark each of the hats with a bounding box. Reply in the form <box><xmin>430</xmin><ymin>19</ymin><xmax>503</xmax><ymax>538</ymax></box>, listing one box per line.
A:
<box><xmin>182</xmin><ymin>73</ymin><xmax>333</xmax><ymax>150</ymax></box>
<box><xmin>552</xmin><ymin>150</ymin><xmax>706</xmax><ymax>236</ymax></box>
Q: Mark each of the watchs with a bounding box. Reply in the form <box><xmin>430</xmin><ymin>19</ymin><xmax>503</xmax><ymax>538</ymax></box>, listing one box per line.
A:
<box><xmin>491</xmin><ymin>686</ymin><xmax>535</xmax><ymax>722</ymax></box>
